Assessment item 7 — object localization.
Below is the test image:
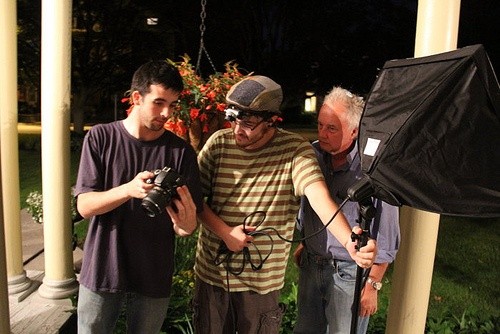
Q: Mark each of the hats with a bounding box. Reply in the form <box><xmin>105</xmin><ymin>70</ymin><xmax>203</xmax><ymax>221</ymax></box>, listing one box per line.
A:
<box><xmin>226</xmin><ymin>75</ymin><xmax>283</xmax><ymax>113</ymax></box>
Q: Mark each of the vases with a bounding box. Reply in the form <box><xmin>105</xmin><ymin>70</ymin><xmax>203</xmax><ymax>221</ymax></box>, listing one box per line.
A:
<box><xmin>188</xmin><ymin>108</ymin><xmax>228</xmax><ymax>151</ymax></box>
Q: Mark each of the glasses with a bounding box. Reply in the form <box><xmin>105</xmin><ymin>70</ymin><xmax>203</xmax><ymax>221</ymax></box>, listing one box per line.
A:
<box><xmin>234</xmin><ymin>119</ymin><xmax>266</xmax><ymax>131</ymax></box>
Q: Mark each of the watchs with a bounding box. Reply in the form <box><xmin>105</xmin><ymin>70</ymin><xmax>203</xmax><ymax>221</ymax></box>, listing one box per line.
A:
<box><xmin>367</xmin><ymin>277</ymin><xmax>382</xmax><ymax>290</ymax></box>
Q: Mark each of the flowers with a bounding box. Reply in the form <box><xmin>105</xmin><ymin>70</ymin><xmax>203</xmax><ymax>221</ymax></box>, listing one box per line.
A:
<box><xmin>121</xmin><ymin>54</ymin><xmax>283</xmax><ymax>137</ymax></box>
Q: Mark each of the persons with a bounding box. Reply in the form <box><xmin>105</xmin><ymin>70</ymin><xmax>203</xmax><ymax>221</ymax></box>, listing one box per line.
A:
<box><xmin>294</xmin><ymin>87</ymin><xmax>400</xmax><ymax>333</ymax></box>
<box><xmin>74</xmin><ymin>61</ymin><xmax>204</xmax><ymax>334</ymax></box>
<box><xmin>197</xmin><ymin>76</ymin><xmax>376</xmax><ymax>334</ymax></box>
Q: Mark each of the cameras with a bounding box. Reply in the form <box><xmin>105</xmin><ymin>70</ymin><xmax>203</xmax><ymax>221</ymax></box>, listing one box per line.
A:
<box><xmin>139</xmin><ymin>166</ymin><xmax>186</xmax><ymax>219</ymax></box>
